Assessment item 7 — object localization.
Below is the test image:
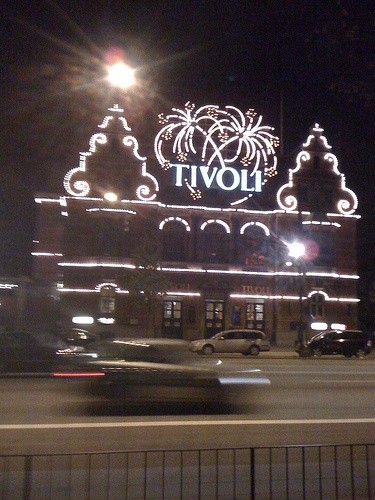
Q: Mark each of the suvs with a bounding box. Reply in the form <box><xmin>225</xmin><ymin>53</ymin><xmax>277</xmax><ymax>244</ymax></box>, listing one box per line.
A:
<box><xmin>295</xmin><ymin>329</ymin><xmax>371</xmax><ymax>358</ymax></box>
<box><xmin>188</xmin><ymin>329</ymin><xmax>270</xmax><ymax>358</ymax></box>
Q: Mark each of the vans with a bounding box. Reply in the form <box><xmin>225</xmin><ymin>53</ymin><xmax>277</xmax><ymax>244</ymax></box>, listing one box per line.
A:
<box><xmin>0</xmin><ymin>330</ymin><xmax>75</xmax><ymax>372</ymax></box>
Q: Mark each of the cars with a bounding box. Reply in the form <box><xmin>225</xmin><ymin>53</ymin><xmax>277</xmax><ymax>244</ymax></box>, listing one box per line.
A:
<box><xmin>53</xmin><ymin>339</ymin><xmax>270</xmax><ymax>415</ymax></box>
